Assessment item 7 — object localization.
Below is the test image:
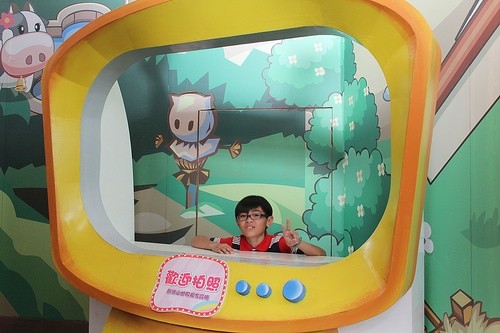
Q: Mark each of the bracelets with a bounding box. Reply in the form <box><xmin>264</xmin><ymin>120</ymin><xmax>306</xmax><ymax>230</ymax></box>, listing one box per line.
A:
<box><xmin>290</xmin><ymin>237</ymin><xmax>303</xmax><ymax>250</ymax></box>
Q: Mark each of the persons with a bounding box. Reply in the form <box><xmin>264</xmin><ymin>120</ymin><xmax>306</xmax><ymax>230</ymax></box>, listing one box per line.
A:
<box><xmin>191</xmin><ymin>196</ymin><xmax>326</xmax><ymax>256</ymax></box>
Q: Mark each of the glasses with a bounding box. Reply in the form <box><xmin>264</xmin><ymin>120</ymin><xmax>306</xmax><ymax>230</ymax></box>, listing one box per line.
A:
<box><xmin>236</xmin><ymin>210</ymin><xmax>268</xmax><ymax>221</ymax></box>
